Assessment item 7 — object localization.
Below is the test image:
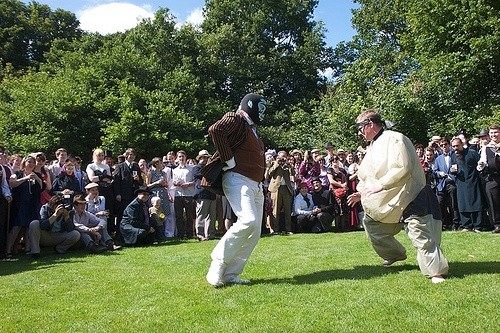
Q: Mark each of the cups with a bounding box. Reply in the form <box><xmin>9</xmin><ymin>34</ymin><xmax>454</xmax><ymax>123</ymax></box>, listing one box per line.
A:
<box><xmin>133</xmin><ymin>171</ymin><xmax>137</xmax><ymax>177</ymax></box>
<box><xmin>160</xmin><ymin>177</ymin><xmax>164</xmax><ymax>183</ymax></box>
<box><xmin>452</xmin><ymin>164</ymin><xmax>458</xmax><ymax>171</ymax></box>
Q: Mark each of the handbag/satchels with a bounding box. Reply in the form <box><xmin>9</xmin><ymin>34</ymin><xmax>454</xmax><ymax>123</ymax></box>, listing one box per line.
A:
<box><xmin>268</xmin><ymin>176</ymin><xmax>281</xmax><ymax>193</ymax></box>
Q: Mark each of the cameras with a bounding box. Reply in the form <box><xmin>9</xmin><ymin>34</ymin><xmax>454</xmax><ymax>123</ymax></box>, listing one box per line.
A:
<box><xmin>60</xmin><ymin>194</ymin><xmax>74</xmax><ymax>215</ymax></box>
<box><xmin>278</xmin><ymin>156</ymin><xmax>291</xmax><ymax>170</ymax></box>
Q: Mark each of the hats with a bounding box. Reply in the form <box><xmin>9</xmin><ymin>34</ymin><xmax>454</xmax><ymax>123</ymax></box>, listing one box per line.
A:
<box><xmin>475</xmin><ymin>129</ymin><xmax>489</xmax><ymax>137</ymax></box>
<box><xmin>73</xmin><ymin>195</ymin><xmax>87</xmax><ymax>204</ymax></box>
<box><xmin>325</xmin><ymin>142</ymin><xmax>336</xmax><ymax>150</ymax></box>
<box><xmin>299</xmin><ymin>182</ymin><xmax>309</xmax><ymax>188</ymax></box>
<box><xmin>104</xmin><ymin>150</ymin><xmax>114</xmax><ymax>159</ymax></box>
<box><xmin>311</xmin><ymin>177</ymin><xmax>322</xmax><ymax>184</ymax></box>
<box><xmin>84</xmin><ymin>183</ymin><xmax>99</xmax><ymax>189</ymax></box>
<box><xmin>163</xmin><ymin>156</ymin><xmax>168</xmax><ymax>163</ymax></box>
<box><xmin>134</xmin><ymin>186</ymin><xmax>154</xmax><ymax>196</ymax></box>
<box><xmin>291</xmin><ymin>149</ymin><xmax>302</xmax><ymax>156</ymax></box>
<box><xmin>432</xmin><ymin>135</ymin><xmax>441</xmax><ymax>140</ymax></box>
<box><xmin>195</xmin><ymin>149</ymin><xmax>212</xmax><ymax>161</ymax></box>
<box><xmin>311</xmin><ymin>149</ymin><xmax>320</xmax><ymax>154</ymax></box>
<box><xmin>337</xmin><ymin>149</ymin><xmax>344</xmax><ymax>154</ymax></box>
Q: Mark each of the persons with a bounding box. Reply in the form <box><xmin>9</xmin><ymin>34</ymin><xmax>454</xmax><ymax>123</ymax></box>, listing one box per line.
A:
<box><xmin>196</xmin><ymin>94</ymin><xmax>268</xmax><ymax>287</ymax></box>
<box><xmin>346</xmin><ymin>110</ymin><xmax>450</xmax><ymax>284</ymax></box>
<box><xmin>0</xmin><ymin>125</ymin><xmax>500</xmax><ymax>260</ymax></box>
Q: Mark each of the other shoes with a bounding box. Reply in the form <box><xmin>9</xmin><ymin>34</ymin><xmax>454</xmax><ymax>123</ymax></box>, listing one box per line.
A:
<box><xmin>2</xmin><ymin>254</ymin><xmax>19</xmax><ymax>262</ymax></box>
<box><xmin>441</xmin><ymin>224</ymin><xmax>500</xmax><ymax>234</ymax></box>
<box><xmin>273</xmin><ymin>231</ymin><xmax>294</xmax><ymax>236</ymax></box>
<box><xmin>108</xmin><ymin>243</ymin><xmax>122</xmax><ymax>251</ymax></box>
<box><xmin>32</xmin><ymin>253</ymin><xmax>40</xmax><ymax>261</ymax></box>
<box><xmin>26</xmin><ymin>251</ymin><xmax>31</xmax><ymax>255</ymax></box>
<box><xmin>90</xmin><ymin>245</ymin><xmax>108</xmax><ymax>255</ymax></box>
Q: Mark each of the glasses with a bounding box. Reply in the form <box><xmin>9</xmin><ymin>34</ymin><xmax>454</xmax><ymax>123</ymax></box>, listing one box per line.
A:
<box><xmin>440</xmin><ymin>143</ymin><xmax>447</xmax><ymax>147</ymax></box>
<box><xmin>356</xmin><ymin>123</ymin><xmax>370</xmax><ymax>132</ymax></box>
<box><xmin>452</xmin><ymin>145</ymin><xmax>459</xmax><ymax>148</ymax></box>
